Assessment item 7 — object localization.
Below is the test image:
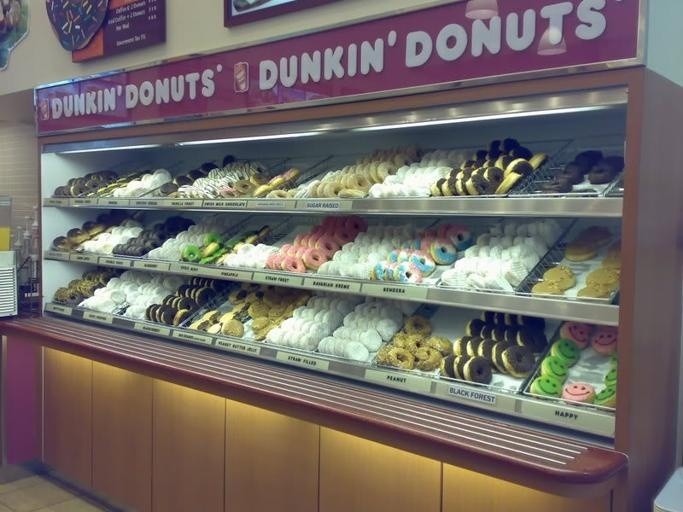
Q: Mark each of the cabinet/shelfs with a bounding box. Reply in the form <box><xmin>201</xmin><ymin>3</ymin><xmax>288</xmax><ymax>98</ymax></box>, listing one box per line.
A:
<box><xmin>36</xmin><ymin>66</ymin><xmax>683</xmax><ymax>457</ymax></box>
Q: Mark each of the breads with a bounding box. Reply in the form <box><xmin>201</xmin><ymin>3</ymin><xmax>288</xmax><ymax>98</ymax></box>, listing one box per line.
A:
<box><xmin>440</xmin><ymin>218</ymin><xmax>622</xmax><ymax>412</ymax></box>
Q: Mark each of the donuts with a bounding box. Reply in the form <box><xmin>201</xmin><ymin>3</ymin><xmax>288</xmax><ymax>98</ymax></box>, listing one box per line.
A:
<box><xmin>53</xmin><ymin>137</ymin><xmax>625</xmax><ymax>198</ymax></box>
<box><xmin>51</xmin><ymin>208</ymin><xmax>547</xmax><ymax>388</ymax></box>
